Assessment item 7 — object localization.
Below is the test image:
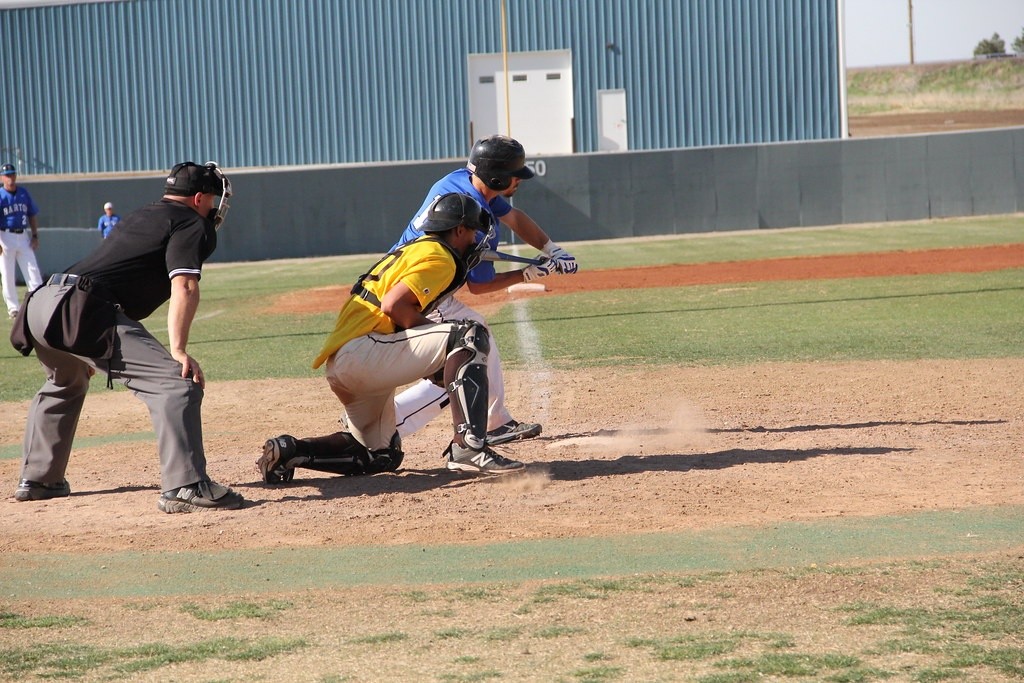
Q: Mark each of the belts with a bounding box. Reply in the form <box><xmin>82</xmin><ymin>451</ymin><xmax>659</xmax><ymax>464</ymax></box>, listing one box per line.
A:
<box><xmin>48</xmin><ymin>272</ymin><xmax>80</xmax><ymax>285</ymax></box>
<box><xmin>0</xmin><ymin>228</ymin><xmax>23</xmax><ymax>233</ymax></box>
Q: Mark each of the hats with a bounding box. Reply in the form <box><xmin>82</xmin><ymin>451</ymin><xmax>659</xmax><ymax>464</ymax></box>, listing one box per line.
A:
<box><xmin>103</xmin><ymin>202</ymin><xmax>112</xmax><ymax>209</ymax></box>
<box><xmin>165</xmin><ymin>163</ymin><xmax>230</xmax><ymax>197</ymax></box>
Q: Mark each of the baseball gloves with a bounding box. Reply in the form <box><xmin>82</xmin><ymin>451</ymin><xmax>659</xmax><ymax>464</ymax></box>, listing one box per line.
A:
<box><xmin>424</xmin><ymin>367</ymin><xmax>445</xmax><ymax>388</ymax></box>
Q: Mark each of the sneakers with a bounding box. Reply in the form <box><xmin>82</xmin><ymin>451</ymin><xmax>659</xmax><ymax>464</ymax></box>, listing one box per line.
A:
<box><xmin>15</xmin><ymin>478</ymin><xmax>71</xmax><ymax>500</ymax></box>
<box><xmin>485</xmin><ymin>419</ymin><xmax>542</xmax><ymax>444</ymax></box>
<box><xmin>258</xmin><ymin>434</ymin><xmax>298</xmax><ymax>484</ymax></box>
<box><xmin>158</xmin><ymin>480</ymin><xmax>244</xmax><ymax>513</ymax></box>
<box><xmin>446</xmin><ymin>442</ymin><xmax>525</xmax><ymax>473</ymax></box>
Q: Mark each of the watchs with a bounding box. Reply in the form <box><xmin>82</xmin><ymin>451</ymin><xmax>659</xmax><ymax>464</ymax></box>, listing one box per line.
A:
<box><xmin>33</xmin><ymin>234</ymin><xmax>38</xmax><ymax>238</ymax></box>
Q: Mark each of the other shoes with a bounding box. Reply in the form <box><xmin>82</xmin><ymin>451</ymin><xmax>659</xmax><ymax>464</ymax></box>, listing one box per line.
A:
<box><xmin>9</xmin><ymin>311</ymin><xmax>19</xmax><ymax>319</ymax></box>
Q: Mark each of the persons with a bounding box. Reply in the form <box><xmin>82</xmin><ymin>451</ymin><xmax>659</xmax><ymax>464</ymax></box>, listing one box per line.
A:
<box><xmin>256</xmin><ymin>192</ymin><xmax>526</xmax><ymax>485</ymax></box>
<box><xmin>11</xmin><ymin>161</ymin><xmax>243</xmax><ymax>515</ymax></box>
<box><xmin>97</xmin><ymin>202</ymin><xmax>119</xmax><ymax>241</ymax></box>
<box><xmin>391</xmin><ymin>136</ymin><xmax>579</xmax><ymax>448</ymax></box>
<box><xmin>0</xmin><ymin>164</ymin><xmax>42</xmax><ymax>318</ymax></box>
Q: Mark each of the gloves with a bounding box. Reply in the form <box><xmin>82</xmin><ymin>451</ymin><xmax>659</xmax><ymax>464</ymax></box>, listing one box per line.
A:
<box><xmin>521</xmin><ymin>254</ymin><xmax>557</xmax><ymax>283</ymax></box>
<box><xmin>548</xmin><ymin>246</ymin><xmax>576</xmax><ymax>275</ymax></box>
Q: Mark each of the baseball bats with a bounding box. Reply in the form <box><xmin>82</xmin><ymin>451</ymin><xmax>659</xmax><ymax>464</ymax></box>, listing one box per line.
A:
<box><xmin>480</xmin><ymin>249</ymin><xmax>578</xmax><ymax>274</ymax></box>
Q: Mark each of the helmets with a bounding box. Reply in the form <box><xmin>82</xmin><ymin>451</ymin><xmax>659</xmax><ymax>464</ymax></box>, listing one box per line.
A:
<box><xmin>0</xmin><ymin>163</ymin><xmax>16</xmax><ymax>174</ymax></box>
<box><xmin>417</xmin><ymin>192</ymin><xmax>484</xmax><ymax>232</ymax></box>
<box><xmin>466</xmin><ymin>135</ymin><xmax>534</xmax><ymax>190</ymax></box>
